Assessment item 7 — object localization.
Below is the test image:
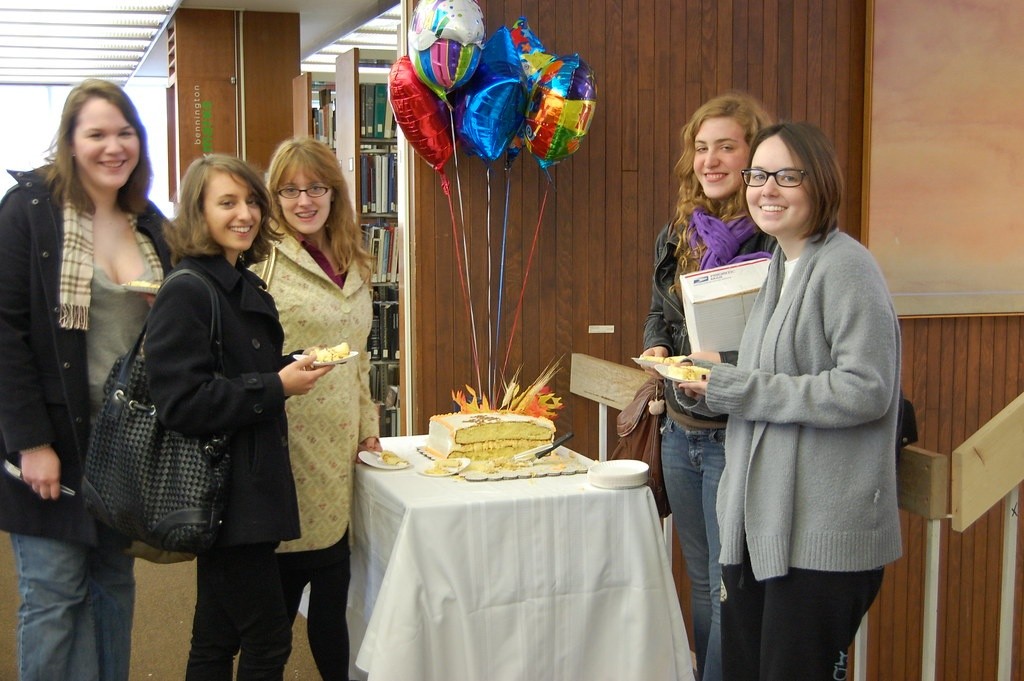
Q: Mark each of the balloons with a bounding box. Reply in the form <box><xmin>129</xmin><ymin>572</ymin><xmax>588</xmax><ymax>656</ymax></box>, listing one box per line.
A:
<box><xmin>387</xmin><ymin>0</ymin><xmax>598</xmax><ymax>195</ymax></box>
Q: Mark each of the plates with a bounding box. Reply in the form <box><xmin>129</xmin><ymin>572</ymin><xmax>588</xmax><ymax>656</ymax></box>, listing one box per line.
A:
<box><xmin>416</xmin><ymin>457</ymin><xmax>470</xmax><ymax>477</ymax></box>
<box><xmin>121</xmin><ymin>285</ymin><xmax>158</xmax><ymax>295</ymax></box>
<box><xmin>654</xmin><ymin>363</ymin><xmax>711</xmax><ymax>382</ymax></box>
<box><xmin>294</xmin><ymin>351</ymin><xmax>359</xmax><ymax>366</ymax></box>
<box><xmin>358</xmin><ymin>450</ymin><xmax>410</xmax><ymax>469</ymax></box>
<box><xmin>587</xmin><ymin>460</ymin><xmax>650</xmax><ymax>488</ymax></box>
<box><xmin>632</xmin><ymin>354</ymin><xmax>669</xmax><ymax>367</ymax></box>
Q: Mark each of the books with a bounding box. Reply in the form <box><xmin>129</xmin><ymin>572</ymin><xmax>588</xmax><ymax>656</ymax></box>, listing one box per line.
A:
<box><xmin>311</xmin><ymin>82</ymin><xmax>401</xmax><ymax>437</ymax></box>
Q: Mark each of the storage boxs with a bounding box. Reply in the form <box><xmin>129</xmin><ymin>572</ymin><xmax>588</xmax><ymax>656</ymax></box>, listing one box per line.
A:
<box><xmin>678</xmin><ymin>258</ymin><xmax>770</xmax><ymax>355</ymax></box>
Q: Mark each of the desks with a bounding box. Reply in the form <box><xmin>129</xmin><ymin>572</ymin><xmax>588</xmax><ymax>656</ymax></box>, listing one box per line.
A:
<box><xmin>296</xmin><ymin>435</ymin><xmax>695</xmax><ymax>681</ymax></box>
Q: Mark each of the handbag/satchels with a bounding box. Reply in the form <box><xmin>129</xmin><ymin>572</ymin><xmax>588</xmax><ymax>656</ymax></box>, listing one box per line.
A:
<box><xmin>80</xmin><ymin>269</ymin><xmax>233</xmax><ymax>555</ymax></box>
<box><xmin>611</xmin><ymin>379</ymin><xmax>672</xmax><ymax>518</ymax></box>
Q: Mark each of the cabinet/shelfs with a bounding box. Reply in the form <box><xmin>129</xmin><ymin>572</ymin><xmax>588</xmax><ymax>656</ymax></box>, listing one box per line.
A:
<box><xmin>292</xmin><ymin>71</ymin><xmax>337</xmax><ymax>152</ymax></box>
<box><xmin>334</xmin><ymin>46</ymin><xmax>401</xmax><ymax>436</ymax></box>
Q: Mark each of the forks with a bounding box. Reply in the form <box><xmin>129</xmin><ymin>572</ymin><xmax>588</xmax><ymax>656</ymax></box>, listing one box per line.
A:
<box><xmin>3</xmin><ymin>460</ymin><xmax>75</xmax><ymax>496</ymax></box>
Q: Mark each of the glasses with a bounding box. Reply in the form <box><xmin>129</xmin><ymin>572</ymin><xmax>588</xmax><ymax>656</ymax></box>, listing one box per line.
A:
<box><xmin>277</xmin><ymin>186</ymin><xmax>332</xmax><ymax>198</ymax></box>
<box><xmin>741</xmin><ymin>168</ymin><xmax>808</xmax><ymax>187</ymax></box>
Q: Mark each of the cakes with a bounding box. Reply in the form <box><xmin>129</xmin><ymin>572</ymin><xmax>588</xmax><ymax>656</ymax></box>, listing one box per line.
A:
<box><xmin>426</xmin><ymin>409</ymin><xmax>557</xmax><ymax>473</ymax></box>
<box><xmin>639</xmin><ymin>354</ymin><xmax>710</xmax><ymax>380</ymax></box>
<box><xmin>313</xmin><ymin>343</ymin><xmax>351</xmax><ymax>364</ymax></box>
<box><xmin>381</xmin><ymin>451</ymin><xmax>401</xmax><ymax>465</ymax></box>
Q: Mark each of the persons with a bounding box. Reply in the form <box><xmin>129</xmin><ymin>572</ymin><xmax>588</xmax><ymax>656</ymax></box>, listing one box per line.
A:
<box><xmin>639</xmin><ymin>97</ymin><xmax>903</xmax><ymax>681</ymax></box>
<box><xmin>245</xmin><ymin>138</ymin><xmax>385</xmax><ymax>681</ymax></box>
<box><xmin>0</xmin><ymin>81</ymin><xmax>175</xmax><ymax>681</ymax></box>
<box><xmin>143</xmin><ymin>154</ymin><xmax>335</xmax><ymax>681</ymax></box>
